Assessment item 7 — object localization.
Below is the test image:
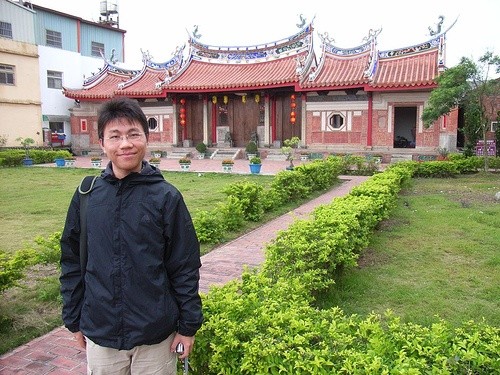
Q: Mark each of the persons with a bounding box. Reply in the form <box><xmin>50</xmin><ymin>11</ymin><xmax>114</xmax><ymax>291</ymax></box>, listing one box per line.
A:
<box><xmin>59</xmin><ymin>98</ymin><xmax>204</xmax><ymax>375</ymax></box>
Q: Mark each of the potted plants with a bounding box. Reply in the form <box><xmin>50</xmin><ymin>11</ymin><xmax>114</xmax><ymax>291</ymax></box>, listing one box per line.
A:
<box><xmin>53</xmin><ymin>152</ymin><xmax>66</xmax><ymax>166</ymax></box>
<box><xmin>222</xmin><ymin>160</ymin><xmax>234</xmax><ymax>171</ymax></box>
<box><xmin>196</xmin><ymin>143</ymin><xmax>206</xmax><ymax>160</ymax></box>
<box><xmin>179</xmin><ymin>159</ymin><xmax>191</xmax><ymax>170</ymax></box>
<box><xmin>151</xmin><ymin>150</ymin><xmax>162</xmax><ymax>157</ymax></box>
<box><xmin>245</xmin><ymin>141</ymin><xmax>257</xmax><ymax>160</ymax></box>
<box><xmin>278</xmin><ymin>136</ymin><xmax>300</xmax><ymax>170</ymax></box>
<box><xmin>149</xmin><ymin>158</ymin><xmax>161</xmax><ymax>167</ymax></box>
<box><xmin>64</xmin><ymin>157</ymin><xmax>77</xmax><ymax>167</ymax></box>
<box><xmin>301</xmin><ymin>153</ymin><xmax>309</xmax><ymax>161</ymax></box>
<box><xmin>15</xmin><ymin>138</ymin><xmax>34</xmax><ymax>165</ymax></box>
<box><xmin>249</xmin><ymin>158</ymin><xmax>262</xmax><ymax>174</ymax></box>
<box><xmin>90</xmin><ymin>157</ymin><xmax>102</xmax><ymax>167</ymax></box>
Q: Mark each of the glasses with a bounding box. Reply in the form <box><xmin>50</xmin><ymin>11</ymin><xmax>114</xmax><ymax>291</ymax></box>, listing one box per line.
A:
<box><xmin>102</xmin><ymin>130</ymin><xmax>145</xmax><ymax>143</ymax></box>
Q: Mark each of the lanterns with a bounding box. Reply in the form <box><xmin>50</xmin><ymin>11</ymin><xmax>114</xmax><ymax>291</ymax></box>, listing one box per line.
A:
<box><xmin>180</xmin><ymin>98</ymin><xmax>186</xmax><ymax>126</ymax></box>
<box><xmin>213</xmin><ymin>96</ymin><xmax>217</xmax><ymax>104</ymax></box>
<box><xmin>255</xmin><ymin>94</ymin><xmax>259</xmax><ymax>103</ymax></box>
<box><xmin>290</xmin><ymin>93</ymin><xmax>296</xmax><ymax>125</ymax></box>
<box><xmin>224</xmin><ymin>96</ymin><xmax>228</xmax><ymax>105</ymax></box>
<box><xmin>242</xmin><ymin>95</ymin><xmax>246</xmax><ymax>104</ymax></box>
